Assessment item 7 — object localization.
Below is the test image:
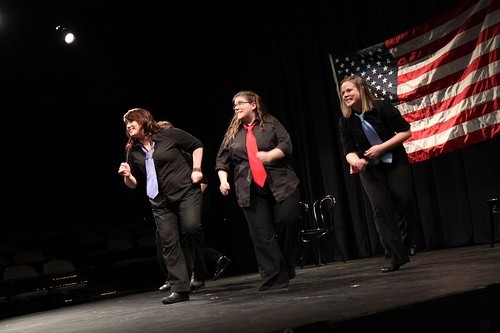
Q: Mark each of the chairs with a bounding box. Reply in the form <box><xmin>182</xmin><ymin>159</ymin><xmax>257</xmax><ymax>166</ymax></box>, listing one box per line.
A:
<box><xmin>486</xmin><ymin>197</ymin><xmax>500</xmax><ymax>248</ymax></box>
<box><xmin>0</xmin><ymin>229</ymin><xmax>159</xmax><ymax>317</ymax></box>
<box><xmin>297</xmin><ymin>195</ymin><xmax>346</xmax><ymax>269</ymax></box>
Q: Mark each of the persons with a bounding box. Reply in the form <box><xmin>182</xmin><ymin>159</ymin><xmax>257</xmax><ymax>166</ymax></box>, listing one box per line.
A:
<box><xmin>215</xmin><ymin>91</ymin><xmax>300</xmax><ymax>290</ymax></box>
<box><xmin>339</xmin><ymin>76</ymin><xmax>417</xmax><ymax>272</ymax></box>
<box><xmin>118</xmin><ymin>108</ymin><xmax>209</xmax><ymax>304</ymax></box>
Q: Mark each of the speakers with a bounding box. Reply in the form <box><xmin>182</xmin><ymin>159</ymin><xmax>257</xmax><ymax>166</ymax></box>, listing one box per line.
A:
<box><xmin>43</xmin><ymin>259</ymin><xmax>77</xmax><ymax>280</ymax></box>
<box><xmin>3</xmin><ymin>264</ymin><xmax>41</xmax><ymax>294</ymax></box>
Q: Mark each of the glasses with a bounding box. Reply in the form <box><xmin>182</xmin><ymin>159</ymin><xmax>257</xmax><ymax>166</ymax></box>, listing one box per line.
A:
<box><xmin>233</xmin><ymin>101</ymin><xmax>253</xmax><ymax>109</ymax></box>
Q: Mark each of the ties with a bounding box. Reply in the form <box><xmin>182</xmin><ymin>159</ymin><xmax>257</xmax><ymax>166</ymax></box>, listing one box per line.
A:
<box><xmin>141</xmin><ymin>142</ymin><xmax>160</xmax><ymax>199</ymax></box>
<box><xmin>355</xmin><ymin>110</ymin><xmax>393</xmax><ymax>164</ymax></box>
<box><xmin>243</xmin><ymin>121</ymin><xmax>267</xmax><ymax>188</ymax></box>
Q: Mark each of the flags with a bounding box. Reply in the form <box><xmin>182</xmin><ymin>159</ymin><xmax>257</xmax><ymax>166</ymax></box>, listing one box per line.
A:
<box><xmin>332</xmin><ymin>0</ymin><xmax>500</xmax><ymax>174</ymax></box>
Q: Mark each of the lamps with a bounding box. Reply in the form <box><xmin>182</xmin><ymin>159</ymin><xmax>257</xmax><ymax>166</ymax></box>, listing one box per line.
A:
<box><xmin>55</xmin><ymin>24</ymin><xmax>75</xmax><ymax>44</ymax></box>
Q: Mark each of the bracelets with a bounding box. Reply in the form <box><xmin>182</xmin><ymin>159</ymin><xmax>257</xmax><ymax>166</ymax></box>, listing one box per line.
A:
<box><xmin>193</xmin><ymin>168</ymin><xmax>201</xmax><ymax>172</ymax></box>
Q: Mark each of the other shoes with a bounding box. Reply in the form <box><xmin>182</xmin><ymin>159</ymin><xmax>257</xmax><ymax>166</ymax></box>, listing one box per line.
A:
<box><xmin>159</xmin><ymin>281</ymin><xmax>171</xmax><ymax>290</ymax></box>
<box><xmin>213</xmin><ymin>256</ymin><xmax>231</xmax><ymax>280</ymax></box>
<box><xmin>162</xmin><ymin>292</ymin><xmax>189</xmax><ymax>304</ymax></box>
<box><xmin>258</xmin><ymin>283</ymin><xmax>288</xmax><ymax>292</ymax></box>
<box><xmin>407</xmin><ymin>241</ymin><xmax>417</xmax><ymax>255</ymax></box>
<box><xmin>381</xmin><ymin>263</ymin><xmax>401</xmax><ymax>272</ymax></box>
<box><xmin>190</xmin><ymin>282</ymin><xmax>205</xmax><ymax>293</ymax></box>
